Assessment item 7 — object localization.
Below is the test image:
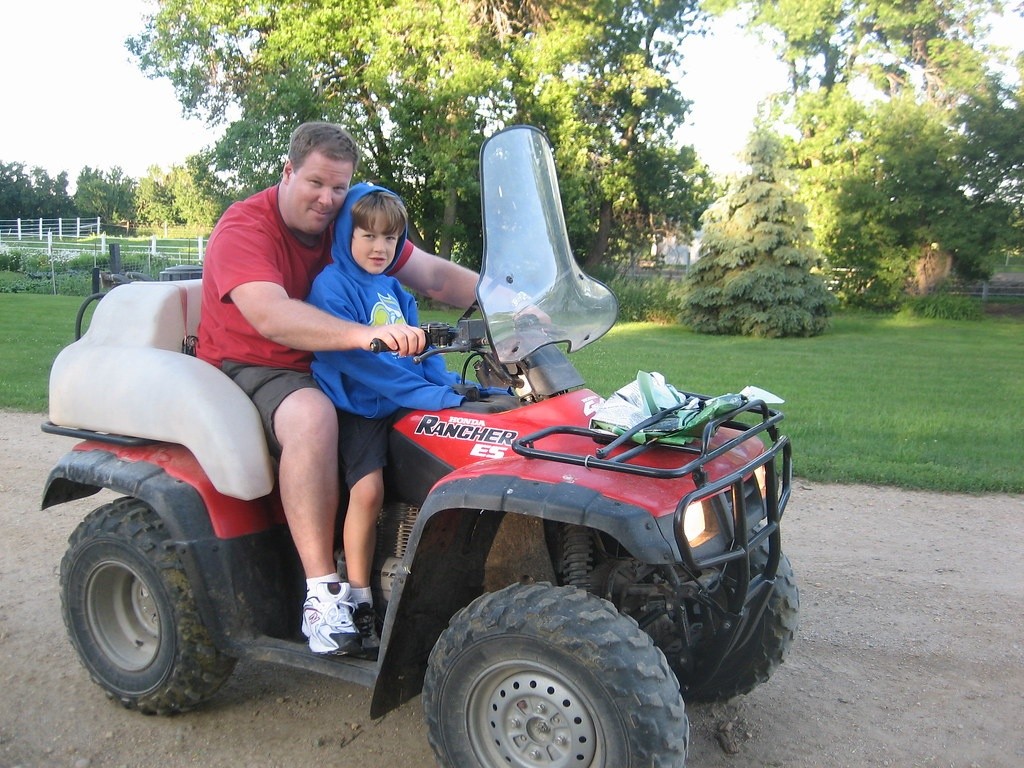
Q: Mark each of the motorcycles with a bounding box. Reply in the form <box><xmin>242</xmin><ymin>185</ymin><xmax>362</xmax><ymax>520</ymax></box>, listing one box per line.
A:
<box><xmin>36</xmin><ymin>126</ymin><xmax>803</xmax><ymax>768</ymax></box>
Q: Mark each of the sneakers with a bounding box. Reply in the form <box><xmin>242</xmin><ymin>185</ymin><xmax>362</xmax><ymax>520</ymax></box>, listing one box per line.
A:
<box><xmin>301</xmin><ymin>582</ymin><xmax>360</xmax><ymax>655</ymax></box>
<box><xmin>353</xmin><ymin>603</ymin><xmax>381</xmax><ymax>655</ymax></box>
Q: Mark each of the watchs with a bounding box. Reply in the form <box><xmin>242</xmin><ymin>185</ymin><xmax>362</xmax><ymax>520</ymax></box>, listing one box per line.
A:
<box><xmin>509</xmin><ymin>290</ymin><xmax>531</xmax><ymax>308</ymax></box>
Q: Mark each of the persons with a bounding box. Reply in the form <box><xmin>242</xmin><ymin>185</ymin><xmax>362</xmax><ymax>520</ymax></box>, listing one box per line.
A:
<box><xmin>198</xmin><ymin>120</ymin><xmax>553</xmax><ymax>658</ymax></box>
<box><xmin>305</xmin><ymin>182</ymin><xmax>507</xmax><ymax>663</ymax></box>
